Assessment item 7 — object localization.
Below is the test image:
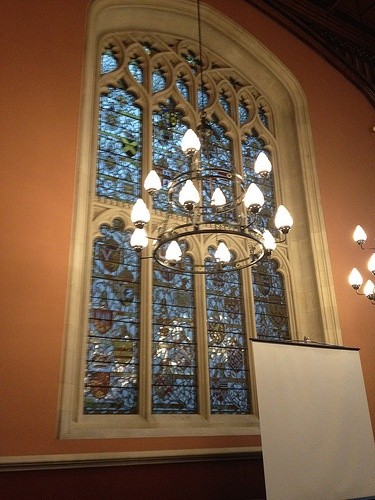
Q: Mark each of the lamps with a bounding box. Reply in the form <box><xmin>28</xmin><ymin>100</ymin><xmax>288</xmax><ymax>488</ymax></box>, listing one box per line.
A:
<box><xmin>129</xmin><ymin>0</ymin><xmax>292</xmax><ymax>275</ymax></box>
<box><xmin>349</xmin><ymin>222</ymin><xmax>375</xmax><ymax>307</ymax></box>
